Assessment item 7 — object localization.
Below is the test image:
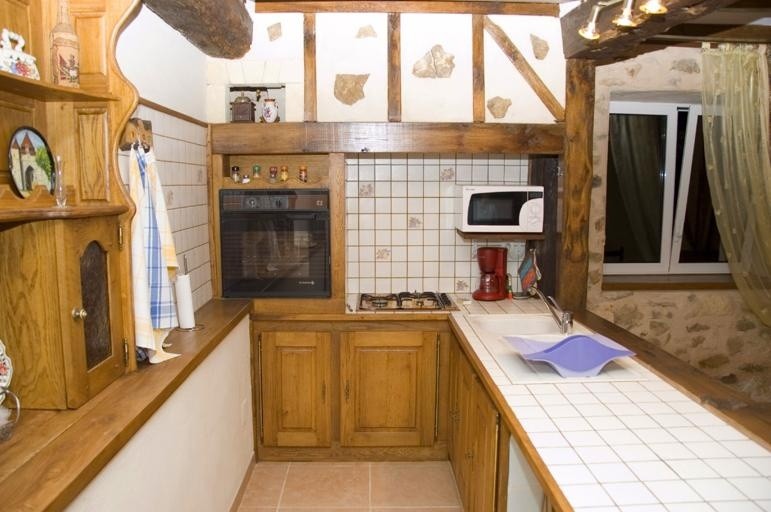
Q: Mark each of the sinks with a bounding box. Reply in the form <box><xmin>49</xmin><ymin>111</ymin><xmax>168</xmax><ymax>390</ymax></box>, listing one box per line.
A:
<box><xmin>462</xmin><ymin>311</ymin><xmax>560</xmax><ymax>338</ymax></box>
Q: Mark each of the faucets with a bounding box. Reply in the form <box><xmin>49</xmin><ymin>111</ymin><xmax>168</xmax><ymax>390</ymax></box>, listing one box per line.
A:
<box><xmin>528</xmin><ymin>284</ymin><xmax>575</xmax><ymax>336</ymax></box>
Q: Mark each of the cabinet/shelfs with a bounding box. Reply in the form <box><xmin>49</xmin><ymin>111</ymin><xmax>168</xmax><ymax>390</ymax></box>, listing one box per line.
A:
<box><xmin>444</xmin><ymin>289</ymin><xmax>771</xmax><ymax>512</ymax></box>
<box><xmin>252</xmin><ymin>312</ymin><xmax>453</xmax><ymax>463</ymax></box>
<box><xmin>1</xmin><ymin>217</ymin><xmax>128</xmax><ymax>411</ymax></box>
<box><xmin>0</xmin><ymin>0</ymin><xmax>139</xmax><ymax>226</ymax></box>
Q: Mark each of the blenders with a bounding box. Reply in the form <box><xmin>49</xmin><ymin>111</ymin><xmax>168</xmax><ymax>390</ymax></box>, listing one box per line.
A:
<box><xmin>473</xmin><ymin>246</ymin><xmax>508</xmax><ymax>300</ymax></box>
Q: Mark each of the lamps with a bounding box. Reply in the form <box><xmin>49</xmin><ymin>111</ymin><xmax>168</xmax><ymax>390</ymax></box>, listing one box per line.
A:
<box><xmin>577</xmin><ymin>0</ymin><xmax>606</xmax><ymax>41</ymax></box>
<box><xmin>612</xmin><ymin>0</ymin><xmax>638</xmax><ymax>28</ymax></box>
<box><xmin>639</xmin><ymin>0</ymin><xmax>669</xmax><ymax>15</ymax></box>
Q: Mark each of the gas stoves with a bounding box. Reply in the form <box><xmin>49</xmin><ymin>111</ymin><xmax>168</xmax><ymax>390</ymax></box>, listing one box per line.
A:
<box><xmin>359</xmin><ymin>291</ymin><xmax>451</xmax><ymax>311</ymax></box>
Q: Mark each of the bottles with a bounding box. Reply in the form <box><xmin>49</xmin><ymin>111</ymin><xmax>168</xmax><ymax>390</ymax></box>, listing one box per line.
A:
<box><xmin>261</xmin><ymin>100</ymin><xmax>279</xmax><ymax>123</ymax></box>
<box><xmin>48</xmin><ymin>5</ymin><xmax>80</xmax><ymax>86</ymax></box>
<box><xmin>229</xmin><ymin>165</ymin><xmax>307</xmax><ymax>183</ymax></box>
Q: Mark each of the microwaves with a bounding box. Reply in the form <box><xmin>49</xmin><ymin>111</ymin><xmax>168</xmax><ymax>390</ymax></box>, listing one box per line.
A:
<box><xmin>463</xmin><ymin>186</ymin><xmax>544</xmax><ymax>234</ymax></box>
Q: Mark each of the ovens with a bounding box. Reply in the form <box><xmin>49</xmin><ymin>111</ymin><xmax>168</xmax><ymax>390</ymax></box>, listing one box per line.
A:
<box><xmin>219</xmin><ymin>188</ymin><xmax>330</xmax><ymax>296</ymax></box>
